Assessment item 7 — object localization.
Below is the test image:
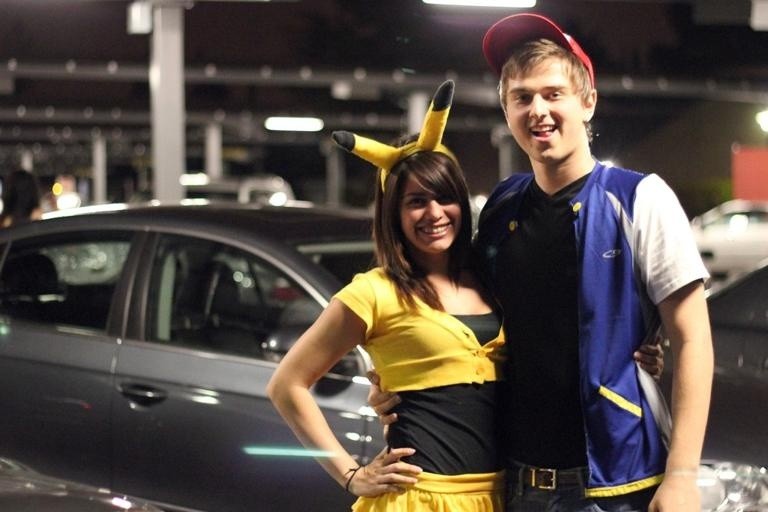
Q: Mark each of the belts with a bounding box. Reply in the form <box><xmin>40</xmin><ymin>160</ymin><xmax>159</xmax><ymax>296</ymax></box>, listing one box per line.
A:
<box><xmin>501</xmin><ymin>465</ymin><xmax>585</xmax><ymax>490</ymax></box>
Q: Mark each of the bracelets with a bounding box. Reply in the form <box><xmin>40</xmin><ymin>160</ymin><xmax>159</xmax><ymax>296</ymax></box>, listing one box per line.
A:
<box><xmin>344</xmin><ymin>465</ymin><xmax>360</xmax><ymax>492</ymax></box>
<box><xmin>663</xmin><ymin>469</ymin><xmax>698</xmax><ymax>477</ymax></box>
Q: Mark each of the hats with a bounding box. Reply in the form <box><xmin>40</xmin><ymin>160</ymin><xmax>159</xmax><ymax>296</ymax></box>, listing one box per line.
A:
<box><xmin>482</xmin><ymin>13</ymin><xmax>594</xmax><ymax>90</ymax></box>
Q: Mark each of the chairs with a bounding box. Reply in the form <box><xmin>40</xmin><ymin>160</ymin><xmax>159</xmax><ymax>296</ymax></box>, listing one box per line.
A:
<box><xmin>1</xmin><ymin>251</ymin><xmax>66</xmax><ymax>326</ymax></box>
<box><xmin>183</xmin><ymin>259</ymin><xmax>261</xmax><ymax>358</ymax></box>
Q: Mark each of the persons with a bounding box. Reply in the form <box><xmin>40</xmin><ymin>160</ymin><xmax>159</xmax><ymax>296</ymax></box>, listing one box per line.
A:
<box><xmin>367</xmin><ymin>12</ymin><xmax>715</xmax><ymax>512</ymax></box>
<box><xmin>267</xmin><ymin>79</ymin><xmax>664</xmax><ymax>512</ymax></box>
<box><xmin>0</xmin><ymin>170</ymin><xmax>42</xmax><ymax>227</ymax></box>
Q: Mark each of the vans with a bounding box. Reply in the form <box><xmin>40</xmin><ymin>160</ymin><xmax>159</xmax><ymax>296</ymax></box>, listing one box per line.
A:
<box><xmin>184</xmin><ymin>172</ymin><xmax>292</xmax><ymax>203</ymax></box>
